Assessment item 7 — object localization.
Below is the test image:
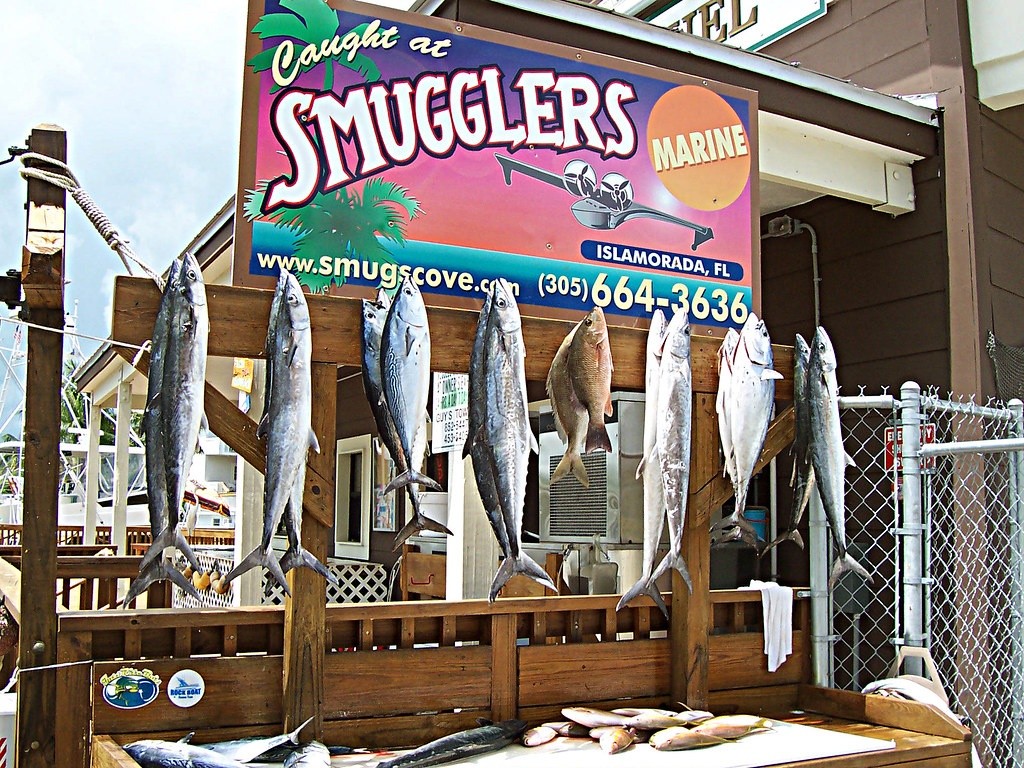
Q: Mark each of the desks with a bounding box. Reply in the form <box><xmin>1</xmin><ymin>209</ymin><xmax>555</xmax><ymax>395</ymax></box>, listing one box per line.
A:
<box><xmin>88</xmin><ymin>683</ymin><xmax>975</xmax><ymax>768</ymax></box>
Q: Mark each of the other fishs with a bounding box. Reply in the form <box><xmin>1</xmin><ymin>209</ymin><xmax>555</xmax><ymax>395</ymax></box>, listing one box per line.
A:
<box><xmin>122</xmin><ymin>706</ymin><xmax>783</xmax><ymax>768</ymax></box>
<box><xmin>121</xmin><ymin>254</ymin><xmax>876</xmax><ymax>625</ymax></box>
